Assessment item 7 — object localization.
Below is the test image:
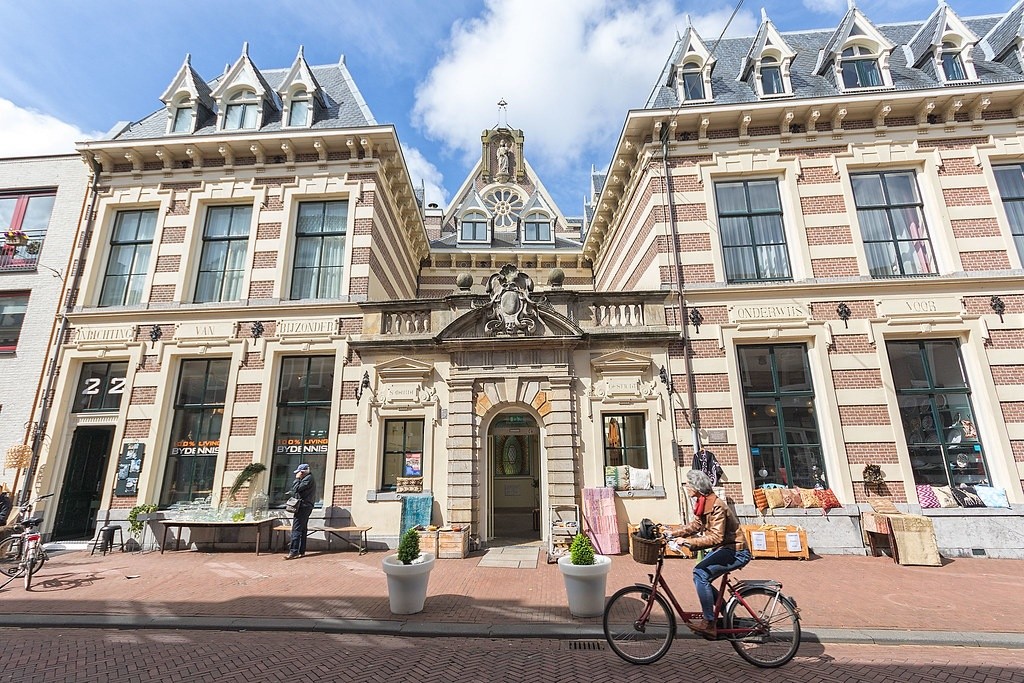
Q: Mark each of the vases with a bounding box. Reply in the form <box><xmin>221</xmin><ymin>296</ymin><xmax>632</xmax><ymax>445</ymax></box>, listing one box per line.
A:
<box><xmin>4</xmin><ymin>236</ymin><xmax>28</xmax><ymax>246</ymax></box>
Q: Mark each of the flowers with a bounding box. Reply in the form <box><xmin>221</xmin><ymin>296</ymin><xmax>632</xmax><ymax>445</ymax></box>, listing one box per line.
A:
<box><xmin>4</xmin><ymin>230</ymin><xmax>29</xmax><ymax>239</ymax></box>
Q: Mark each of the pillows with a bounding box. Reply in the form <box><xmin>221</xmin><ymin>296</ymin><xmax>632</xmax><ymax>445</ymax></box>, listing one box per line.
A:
<box><xmin>604</xmin><ymin>464</ymin><xmax>655</xmax><ymax>492</ymax></box>
<box><xmin>753</xmin><ymin>485</ymin><xmax>843</xmax><ymax>515</ymax></box>
<box><xmin>916</xmin><ymin>482</ymin><xmax>1014</xmax><ymax>513</ymax></box>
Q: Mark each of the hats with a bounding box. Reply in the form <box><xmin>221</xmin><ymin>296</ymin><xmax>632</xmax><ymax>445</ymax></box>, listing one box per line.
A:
<box><xmin>687</xmin><ymin>470</ymin><xmax>712</xmax><ymax>495</ymax></box>
<box><xmin>294</xmin><ymin>464</ymin><xmax>309</xmax><ymax>474</ymax></box>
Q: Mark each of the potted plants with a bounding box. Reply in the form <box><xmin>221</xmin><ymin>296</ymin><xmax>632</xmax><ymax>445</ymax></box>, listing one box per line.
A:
<box><xmin>382</xmin><ymin>528</ymin><xmax>436</xmax><ymax>616</ymax></box>
<box><xmin>559</xmin><ymin>533</ymin><xmax>612</xmax><ymax>618</ymax></box>
<box><xmin>127</xmin><ymin>503</ymin><xmax>164</xmax><ymax>539</ymax></box>
<box><xmin>529</xmin><ymin>479</ymin><xmax>541</xmax><ymax>532</ymax></box>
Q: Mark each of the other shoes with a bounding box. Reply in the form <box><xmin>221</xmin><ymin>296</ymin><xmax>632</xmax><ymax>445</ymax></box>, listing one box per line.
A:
<box><xmin>283</xmin><ymin>553</ymin><xmax>297</xmax><ymax>559</ymax></box>
<box><xmin>293</xmin><ymin>551</ymin><xmax>308</xmax><ymax>558</ymax></box>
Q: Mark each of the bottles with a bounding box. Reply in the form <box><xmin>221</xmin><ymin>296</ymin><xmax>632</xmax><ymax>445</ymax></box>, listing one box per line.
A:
<box><xmin>166</xmin><ymin>488</ymin><xmax>270</xmax><ymax>522</ymax></box>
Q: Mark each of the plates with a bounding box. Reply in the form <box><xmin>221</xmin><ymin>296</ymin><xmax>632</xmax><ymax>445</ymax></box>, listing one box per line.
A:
<box><xmin>947</xmin><ymin>429</ymin><xmax>961</xmax><ymax>443</ymax></box>
<box><xmin>908</xmin><ymin>433</ymin><xmax>922</xmax><ymax>443</ymax></box>
<box><xmin>926</xmin><ymin>433</ymin><xmax>939</xmax><ymax>443</ymax></box>
<box><xmin>900</xmin><ymin>397</ymin><xmax>916</xmax><ymax>414</ymax></box>
<box><xmin>917</xmin><ymin>397</ymin><xmax>931</xmax><ymax>412</ymax></box>
<box><xmin>957</xmin><ymin>453</ymin><xmax>970</xmax><ymax>467</ymax></box>
<box><xmin>952</xmin><ymin>413</ymin><xmax>961</xmax><ymax>426</ymax></box>
<box><xmin>910</xmin><ymin>416</ymin><xmax>921</xmax><ymax>430</ymax></box>
<box><xmin>922</xmin><ymin>416</ymin><xmax>933</xmax><ymax>429</ymax></box>
<box><xmin>935</xmin><ymin>394</ymin><xmax>947</xmax><ymax>409</ymax></box>
<box><xmin>914</xmin><ymin>463</ymin><xmax>944</xmax><ymax>469</ymax></box>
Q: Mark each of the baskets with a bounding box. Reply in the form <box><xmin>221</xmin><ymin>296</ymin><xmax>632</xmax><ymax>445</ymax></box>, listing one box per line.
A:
<box><xmin>630</xmin><ymin>532</ymin><xmax>661</xmax><ymax>565</ymax></box>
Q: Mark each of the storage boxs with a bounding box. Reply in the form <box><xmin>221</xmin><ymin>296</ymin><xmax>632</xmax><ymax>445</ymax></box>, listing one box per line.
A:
<box><xmin>627</xmin><ymin>523</ymin><xmax>810</xmax><ymax>561</ymax></box>
<box><xmin>409</xmin><ymin>524</ymin><xmax>472</xmax><ymax>559</ymax></box>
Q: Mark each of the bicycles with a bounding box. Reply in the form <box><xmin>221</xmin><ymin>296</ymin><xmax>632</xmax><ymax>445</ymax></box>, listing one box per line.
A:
<box><xmin>0</xmin><ymin>493</ymin><xmax>55</xmax><ymax>591</ymax></box>
<box><xmin>603</xmin><ymin>530</ymin><xmax>802</xmax><ymax>669</ymax></box>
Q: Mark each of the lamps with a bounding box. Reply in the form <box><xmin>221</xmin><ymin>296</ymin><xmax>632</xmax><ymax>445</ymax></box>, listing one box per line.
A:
<box><xmin>3</xmin><ymin>419</ymin><xmax>40</xmax><ymax>471</ymax></box>
<box><xmin>748</xmin><ymin>404</ymin><xmax>778</xmax><ymax>418</ymax></box>
<box><xmin>758</xmin><ymin>450</ymin><xmax>769</xmax><ymax>478</ymax></box>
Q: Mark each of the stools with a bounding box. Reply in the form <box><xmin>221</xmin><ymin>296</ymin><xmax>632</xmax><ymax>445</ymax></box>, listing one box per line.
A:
<box><xmin>91</xmin><ymin>524</ymin><xmax>125</xmax><ymax>556</ymax></box>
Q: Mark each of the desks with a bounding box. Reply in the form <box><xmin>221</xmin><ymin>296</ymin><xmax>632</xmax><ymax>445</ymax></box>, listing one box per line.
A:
<box><xmin>861</xmin><ymin>510</ymin><xmax>955</xmax><ymax>568</ymax></box>
<box><xmin>158</xmin><ymin>517</ymin><xmax>280</xmax><ymax>556</ymax></box>
<box><xmin>274</xmin><ymin>524</ymin><xmax>373</xmax><ymax>556</ymax></box>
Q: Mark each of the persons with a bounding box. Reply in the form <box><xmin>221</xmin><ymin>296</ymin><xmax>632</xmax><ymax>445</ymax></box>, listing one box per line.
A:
<box><xmin>283</xmin><ymin>464</ymin><xmax>316</xmax><ymax>560</ymax></box>
<box><xmin>662</xmin><ymin>469</ymin><xmax>751</xmax><ymax>640</ymax></box>
<box><xmin>497</xmin><ymin>140</ymin><xmax>509</xmax><ymax>173</ymax></box>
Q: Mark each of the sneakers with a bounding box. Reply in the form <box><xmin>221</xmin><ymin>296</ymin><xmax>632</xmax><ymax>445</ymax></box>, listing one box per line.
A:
<box><xmin>686</xmin><ymin>620</ymin><xmax>716</xmax><ymax>639</ymax></box>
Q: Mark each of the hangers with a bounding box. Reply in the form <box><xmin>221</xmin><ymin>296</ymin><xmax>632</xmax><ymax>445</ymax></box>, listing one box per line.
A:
<box><xmin>608</xmin><ymin>415</ymin><xmax>620</xmax><ymax>427</ymax></box>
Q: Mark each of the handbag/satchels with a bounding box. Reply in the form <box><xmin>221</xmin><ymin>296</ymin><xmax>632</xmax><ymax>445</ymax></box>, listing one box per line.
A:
<box><xmin>636</xmin><ymin>518</ymin><xmax>662</xmax><ymax>539</ymax></box>
<box><xmin>286</xmin><ymin>497</ymin><xmax>300</xmax><ymax>513</ymax></box>
